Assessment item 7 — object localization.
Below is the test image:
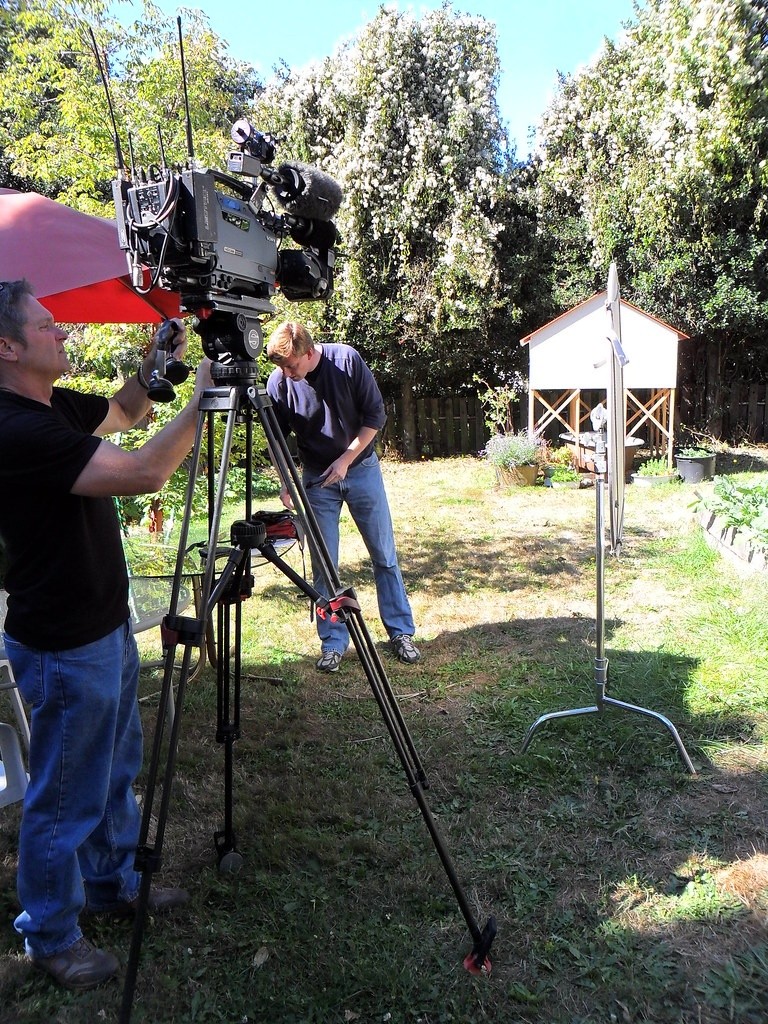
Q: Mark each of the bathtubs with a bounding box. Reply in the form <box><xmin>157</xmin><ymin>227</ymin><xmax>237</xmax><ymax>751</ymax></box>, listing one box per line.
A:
<box><xmin>558</xmin><ymin>430</ymin><xmax>645</xmax><ymax>473</ymax></box>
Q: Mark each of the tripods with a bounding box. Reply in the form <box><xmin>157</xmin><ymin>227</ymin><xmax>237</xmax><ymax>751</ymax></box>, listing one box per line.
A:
<box><xmin>118</xmin><ymin>289</ymin><xmax>500</xmax><ymax>1024</ymax></box>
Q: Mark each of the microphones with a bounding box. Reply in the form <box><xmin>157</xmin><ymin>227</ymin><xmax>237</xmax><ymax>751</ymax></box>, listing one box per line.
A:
<box><xmin>260</xmin><ymin>160</ymin><xmax>343</xmax><ymax>222</ymax></box>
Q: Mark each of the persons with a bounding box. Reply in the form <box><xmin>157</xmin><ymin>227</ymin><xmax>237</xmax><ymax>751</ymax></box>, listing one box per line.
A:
<box><xmin>0</xmin><ymin>279</ymin><xmax>215</xmax><ymax>988</ymax></box>
<box><xmin>266</xmin><ymin>322</ymin><xmax>422</xmax><ymax>670</ymax></box>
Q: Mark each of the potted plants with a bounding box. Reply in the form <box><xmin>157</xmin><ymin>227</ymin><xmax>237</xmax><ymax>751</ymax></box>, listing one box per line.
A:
<box><xmin>477</xmin><ymin>427</ymin><xmax>549</xmax><ymax>488</ymax></box>
<box><xmin>630</xmin><ymin>458</ymin><xmax>680</xmax><ymax>487</ymax></box>
<box><xmin>674</xmin><ymin>446</ymin><xmax>717</xmax><ymax>484</ymax></box>
<box><xmin>541</xmin><ymin>446</ymin><xmax>579</xmax><ymax>477</ymax></box>
<box><xmin>549</xmin><ymin>470</ymin><xmax>583</xmax><ymax>490</ymax></box>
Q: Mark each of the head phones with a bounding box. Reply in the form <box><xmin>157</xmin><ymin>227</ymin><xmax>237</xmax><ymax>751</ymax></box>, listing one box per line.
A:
<box><xmin>146</xmin><ymin>321</ymin><xmax>189</xmax><ymax>403</ymax></box>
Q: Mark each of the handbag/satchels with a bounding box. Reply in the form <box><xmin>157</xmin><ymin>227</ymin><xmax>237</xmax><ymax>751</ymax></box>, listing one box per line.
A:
<box><xmin>252</xmin><ymin>509</ymin><xmax>298</xmax><ymax>538</ymax></box>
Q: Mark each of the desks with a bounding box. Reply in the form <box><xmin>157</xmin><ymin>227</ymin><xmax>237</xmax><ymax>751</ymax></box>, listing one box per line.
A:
<box><xmin>122</xmin><ymin>527</ymin><xmax>297</xmax><ymax>703</ymax></box>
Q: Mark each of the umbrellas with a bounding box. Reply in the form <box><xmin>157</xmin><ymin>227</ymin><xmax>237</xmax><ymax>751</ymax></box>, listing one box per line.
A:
<box><xmin>0</xmin><ymin>189</ymin><xmax>204</xmax><ymax>324</ymax></box>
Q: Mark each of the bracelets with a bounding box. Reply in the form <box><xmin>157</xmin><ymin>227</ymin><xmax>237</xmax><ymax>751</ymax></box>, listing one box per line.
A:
<box><xmin>140</xmin><ymin>364</ymin><xmax>150</xmax><ymax>387</ymax></box>
<box><xmin>137</xmin><ymin>366</ymin><xmax>148</xmax><ymax>389</ymax></box>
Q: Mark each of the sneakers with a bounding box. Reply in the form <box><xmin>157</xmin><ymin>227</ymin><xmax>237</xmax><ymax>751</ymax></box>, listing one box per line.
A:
<box><xmin>104</xmin><ymin>886</ymin><xmax>189</xmax><ymax>916</ymax></box>
<box><xmin>388</xmin><ymin>634</ymin><xmax>420</xmax><ymax>663</ymax></box>
<box><xmin>25</xmin><ymin>937</ymin><xmax>119</xmax><ymax>991</ymax></box>
<box><xmin>316</xmin><ymin>651</ymin><xmax>342</xmax><ymax>672</ymax></box>
